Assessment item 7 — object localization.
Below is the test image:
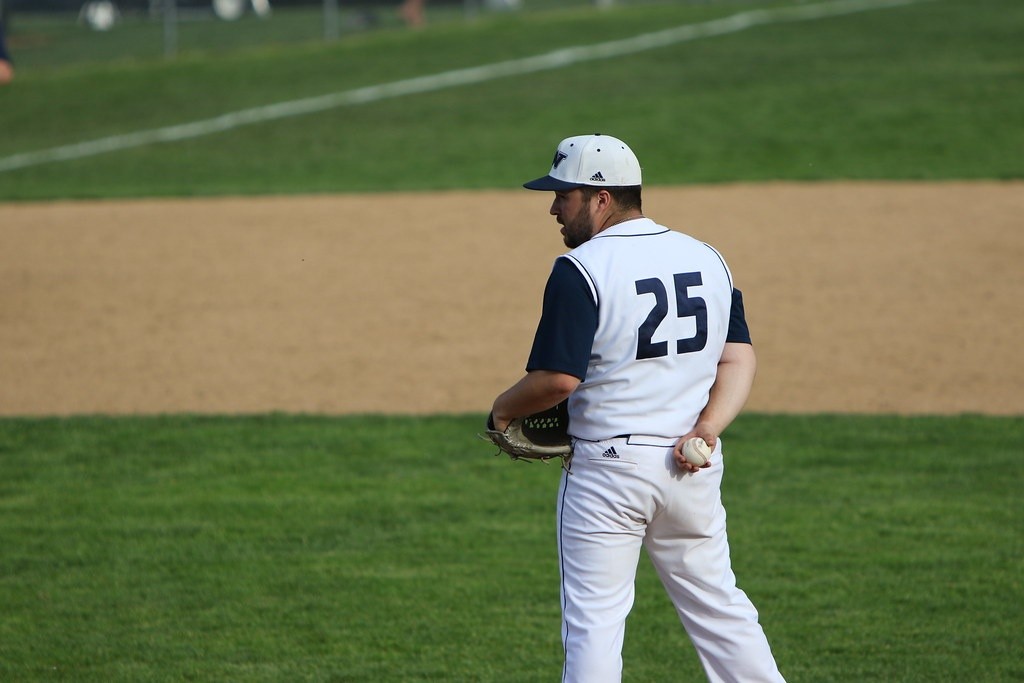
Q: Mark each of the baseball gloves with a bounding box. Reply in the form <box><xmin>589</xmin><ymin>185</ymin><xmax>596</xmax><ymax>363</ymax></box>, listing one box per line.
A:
<box><xmin>476</xmin><ymin>395</ymin><xmax>575</xmax><ymax>478</ymax></box>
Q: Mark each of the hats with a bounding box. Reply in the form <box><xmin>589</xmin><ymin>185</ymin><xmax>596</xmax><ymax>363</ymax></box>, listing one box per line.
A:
<box><xmin>523</xmin><ymin>133</ymin><xmax>642</xmax><ymax>191</ymax></box>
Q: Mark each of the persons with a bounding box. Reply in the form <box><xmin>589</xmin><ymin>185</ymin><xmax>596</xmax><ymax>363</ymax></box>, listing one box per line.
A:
<box><xmin>487</xmin><ymin>133</ymin><xmax>788</xmax><ymax>683</ymax></box>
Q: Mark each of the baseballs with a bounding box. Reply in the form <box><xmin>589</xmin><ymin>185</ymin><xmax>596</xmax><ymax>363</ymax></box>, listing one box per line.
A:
<box><xmin>682</xmin><ymin>436</ymin><xmax>713</xmax><ymax>468</ymax></box>
